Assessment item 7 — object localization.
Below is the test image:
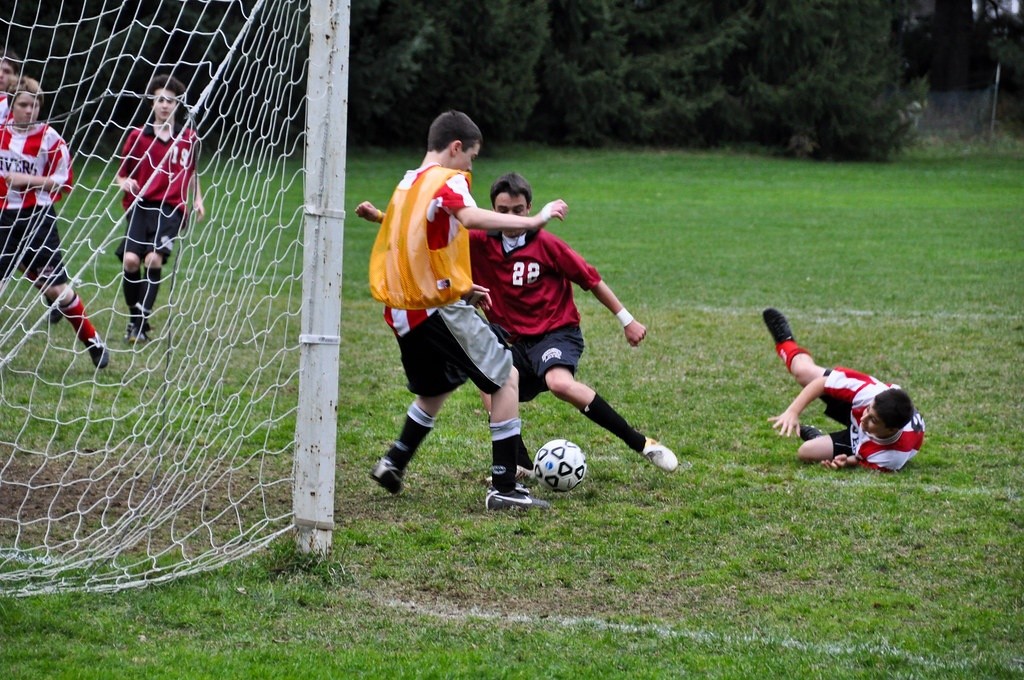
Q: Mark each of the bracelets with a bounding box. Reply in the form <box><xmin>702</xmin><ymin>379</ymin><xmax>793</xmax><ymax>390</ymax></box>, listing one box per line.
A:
<box><xmin>616</xmin><ymin>308</ymin><xmax>634</xmax><ymax>329</ymax></box>
<box><xmin>374</xmin><ymin>209</ymin><xmax>383</xmax><ymax>222</ymax></box>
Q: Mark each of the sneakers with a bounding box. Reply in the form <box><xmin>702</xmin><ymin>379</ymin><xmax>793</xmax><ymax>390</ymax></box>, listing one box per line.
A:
<box><xmin>371</xmin><ymin>456</ymin><xmax>402</xmax><ymax>495</ymax></box>
<box><xmin>47</xmin><ymin>297</ymin><xmax>63</xmax><ymax>323</ymax></box>
<box><xmin>800</xmin><ymin>425</ymin><xmax>821</xmax><ymax>438</ymax></box>
<box><xmin>486</xmin><ymin>459</ymin><xmax>535</xmax><ymax>485</ymax></box>
<box><xmin>84</xmin><ymin>332</ymin><xmax>109</xmax><ymax>368</ymax></box>
<box><xmin>485</xmin><ymin>483</ymin><xmax>551</xmax><ymax>509</ymax></box>
<box><xmin>638</xmin><ymin>437</ymin><xmax>678</xmax><ymax>472</ymax></box>
<box><xmin>765</xmin><ymin>308</ymin><xmax>794</xmax><ymax>341</ymax></box>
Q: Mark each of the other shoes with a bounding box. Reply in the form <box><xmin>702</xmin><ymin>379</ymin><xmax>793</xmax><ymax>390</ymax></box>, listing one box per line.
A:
<box><xmin>124</xmin><ymin>323</ymin><xmax>133</xmax><ymax>340</ymax></box>
<box><xmin>129</xmin><ymin>327</ymin><xmax>145</xmax><ymax>343</ymax></box>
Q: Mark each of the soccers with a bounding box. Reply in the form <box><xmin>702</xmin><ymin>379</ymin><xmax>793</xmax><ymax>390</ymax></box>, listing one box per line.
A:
<box><xmin>532</xmin><ymin>438</ymin><xmax>586</xmax><ymax>493</ymax></box>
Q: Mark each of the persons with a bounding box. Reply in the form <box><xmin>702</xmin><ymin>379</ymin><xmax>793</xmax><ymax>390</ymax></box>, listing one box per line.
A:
<box><xmin>369</xmin><ymin>111</ymin><xmax>569</xmax><ymax>517</ymax></box>
<box><xmin>0</xmin><ymin>57</ymin><xmax>110</xmax><ymax>368</ymax></box>
<box><xmin>113</xmin><ymin>75</ymin><xmax>207</xmax><ymax>343</ymax></box>
<box><xmin>354</xmin><ymin>173</ymin><xmax>677</xmax><ymax>485</ymax></box>
<box><xmin>762</xmin><ymin>308</ymin><xmax>925</xmax><ymax>472</ymax></box>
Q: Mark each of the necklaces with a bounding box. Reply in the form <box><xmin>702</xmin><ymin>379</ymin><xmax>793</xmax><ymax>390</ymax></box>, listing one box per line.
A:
<box><xmin>503</xmin><ymin>229</ymin><xmax>526</xmax><ymax>247</ymax></box>
